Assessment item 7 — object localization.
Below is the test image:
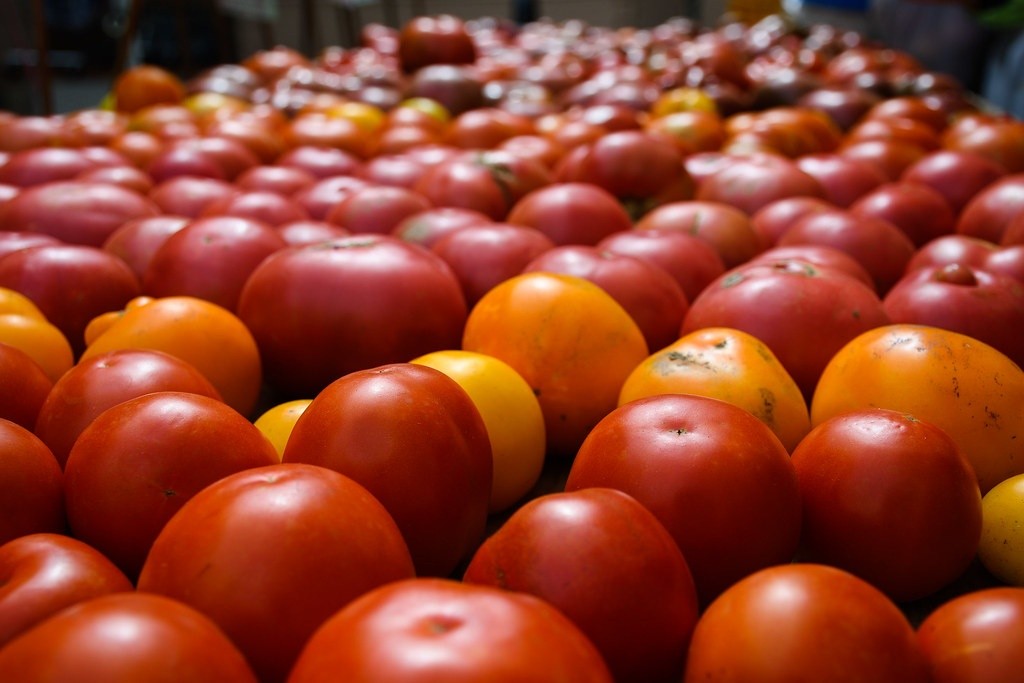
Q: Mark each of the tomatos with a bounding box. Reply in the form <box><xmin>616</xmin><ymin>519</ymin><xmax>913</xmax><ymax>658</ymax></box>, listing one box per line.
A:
<box><xmin>0</xmin><ymin>15</ymin><xmax>1024</xmax><ymax>683</ymax></box>
